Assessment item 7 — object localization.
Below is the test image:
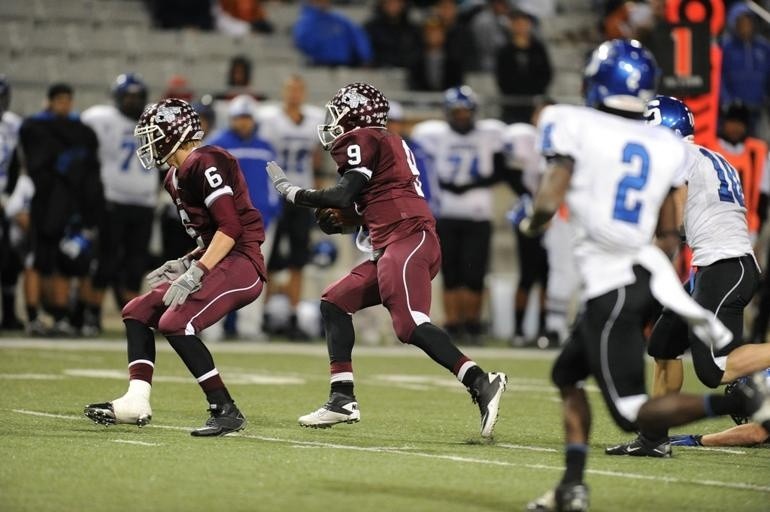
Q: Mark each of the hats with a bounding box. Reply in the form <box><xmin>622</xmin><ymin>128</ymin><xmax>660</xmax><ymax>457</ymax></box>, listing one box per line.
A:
<box><xmin>226</xmin><ymin>94</ymin><xmax>260</xmax><ymax>120</ymax></box>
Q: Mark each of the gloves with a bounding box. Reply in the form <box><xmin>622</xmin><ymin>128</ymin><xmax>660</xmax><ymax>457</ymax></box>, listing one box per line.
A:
<box><xmin>161</xmin><ymin>259</ymin><xmax>210</xmax><ymax>308</ymax></box>
<box><xmin>265</xmin><ymin>159</ymin><xmax>302</xmax><ymax>205</ymax></box>
<box><xmin>504</xmin><ymin>193</ymin><xmax>541</xmax><ymax>239</ymax></box>
<box><xmin>145</xmin><ymin>257</ymin><xmax>192</xmax><ymax>286</ymax></box>
<box><xmin>668</xmin><ymin>433</ymin><xmax>704</xmax><ymax>449</ymax></box>
<box><xmin>313</xmin><ymin>206</ymin><xmax>344</xmax><ymax>236</ymax></box>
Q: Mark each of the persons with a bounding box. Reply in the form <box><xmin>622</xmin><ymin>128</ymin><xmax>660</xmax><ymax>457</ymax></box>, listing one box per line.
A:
<box><xmin>669</xmin><ymin>367</ymin><xmax>770</xmax><ymax>452</ymax></box>
<box><xmin>143</xmin><ymin>2</ymin><xmax>557</xmax><ymax>127</ymax></box>
<box><xmin>501</xmin><ymin>32</ymin><xmax>769</xmax><ymax>510</ymax></box>
<box><xmin>1</xmin><ymin>74</ymin><xmax>199</xmax><ymax>338</ymax></box>
<box><xmin>195</xmin><ymin>55</ymin><xmax>561</xmax><ymax>350</ymax></box>
<box><xmin>262</xmin><ymin>80</ymin><xmax>510</xmax><ymax>444</ymax></box>
<box><xmin>602</xmin><ymin>92</ymin><xmax>764</xmax><ymax>460</ymax></box>
<box><xmin>79</xmin><ymin>93</ymin><xmax>270</xmax><ymax>438</ymax></box>
<box><xmin>558</xmin><ymin>2</ymin><xmax>769</xmax><ymax>352</ymax></box>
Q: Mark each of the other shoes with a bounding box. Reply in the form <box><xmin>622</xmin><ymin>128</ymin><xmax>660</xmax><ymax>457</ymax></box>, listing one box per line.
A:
<box><xmin>0</xmin><ymin>310</ymin><xmax>101</xmax><ymax>339</ymax></box>
<box><xmin>222</xmin><ymin>310</ymin><xmax>312</xmax><ymax>343</ymax></box>
<box><xmin>441</xmin><ymin>317</ymin><xmax>555</xmax><ymax>348</ymax></box>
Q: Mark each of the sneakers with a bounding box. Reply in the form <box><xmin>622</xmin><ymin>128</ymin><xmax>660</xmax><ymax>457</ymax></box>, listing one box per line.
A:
<box><xmin>465</xmin><ymin>368</ymin><xmax>508</xmax><ymax>440</ymax></box>
<box><xmin>527</xmin><ymin>483</ymin><xmax>597</xmax><ymax>511</ymax></box>
<box><xmin>85</xmin><ymin>390</ymin><xmax>153</xmax><ymax>428</ymax></box>
<box><xmin>603</xmin><ymin>428</ymin><xmax>672</xmax><ymax>460</ymax></box>
<box><xmin>298</xmin><ymin>389</ymin><xmax>362</xmax><ymax>429</ymax></box>
<box><xmin>192</xmin><ymin>400</ymin><xmax>249</xmax><ymax>440</ymax></box>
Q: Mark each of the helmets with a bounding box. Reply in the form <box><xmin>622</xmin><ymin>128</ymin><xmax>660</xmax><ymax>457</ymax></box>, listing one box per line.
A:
<box><xmin>325</xmin><ymin>81</ymin><xmax>391</xmax><ymax>135</ymax></box>
<box><xmin>444</xmin><ymin>85</ymin><xmax>476</xmax><ymax>124</ymax></box>
<box><xmin>581</xmin><ymin>34</ymin><xmax>663</xmax><ymax>116</ymax></box>
<box><xmin>108</xmin><ymin>72</ymin><xmax>149</xmax><ymax>111</ymax></box>
<box><xmin>135</xmin><ymin>97</ymin><xmax>205</xmax><ymax>167</ymax></box>
<box><xmin>641</xmin><ymin>94</ymin><xmax>699</xmax><ymax>144</ymax></box>
